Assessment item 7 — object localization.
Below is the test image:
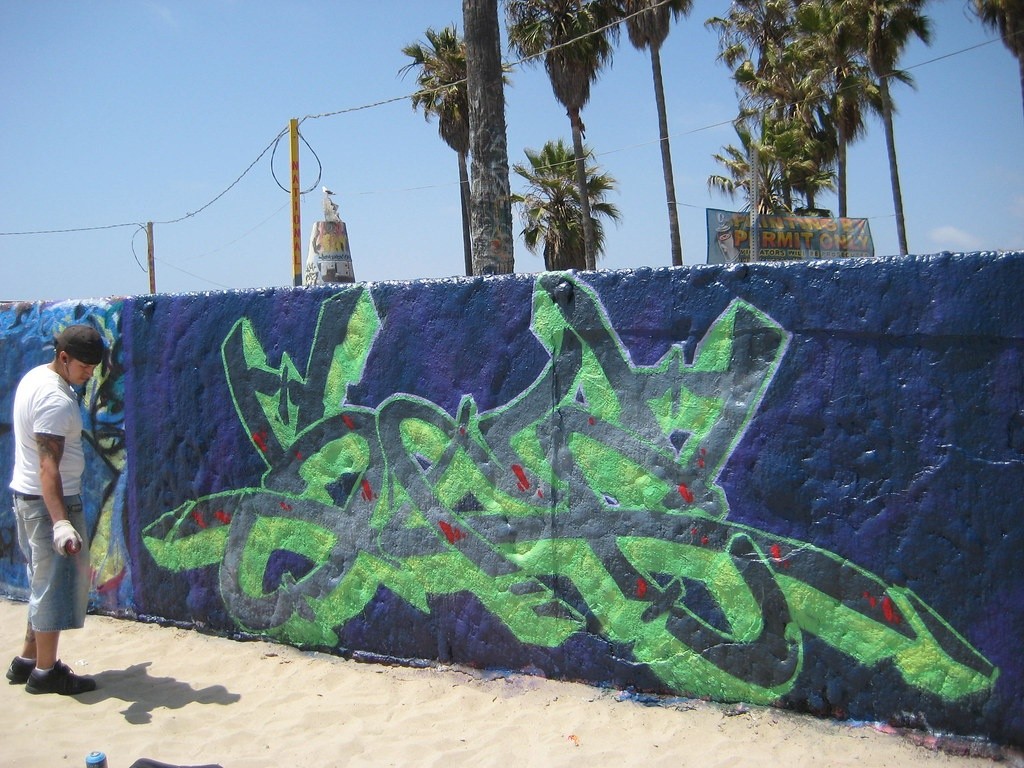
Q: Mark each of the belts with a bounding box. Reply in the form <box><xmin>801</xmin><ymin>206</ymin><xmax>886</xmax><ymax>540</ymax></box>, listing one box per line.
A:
<box><xmin>12</xmin><ymin>490</ymin><xmax>40</xmax><ymax>501</ymax></box>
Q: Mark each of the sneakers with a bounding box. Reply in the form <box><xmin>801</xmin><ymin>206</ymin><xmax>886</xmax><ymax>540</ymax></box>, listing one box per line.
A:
<box><xmin>25</xmin><ymin>658</ymin><xmax>96</xmax><ymax>696</ymax></box>
<box><xmin>6</xmin><ymin>655</ymin><xmax>36</xmax><ymax>682</ymax></box>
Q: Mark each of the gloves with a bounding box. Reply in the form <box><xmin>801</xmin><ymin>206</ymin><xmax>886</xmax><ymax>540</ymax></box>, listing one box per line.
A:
<box><xmin>52</xmin><ymin>520</ymin><xmax>83</xmax><ymax>558</ymax></box>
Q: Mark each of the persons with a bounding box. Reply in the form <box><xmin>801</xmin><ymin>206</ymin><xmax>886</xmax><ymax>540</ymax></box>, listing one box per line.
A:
<box><xmin>6</xmin><ymin>325</ymin><xmax>105</xmax><ymax>697</ymax></box>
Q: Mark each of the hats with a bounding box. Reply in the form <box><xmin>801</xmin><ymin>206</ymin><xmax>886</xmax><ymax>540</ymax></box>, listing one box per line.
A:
<box><xmin>56</xmin><ymin>325</ymin><xmax>103</xmax><ymax>364</ymax></box>
